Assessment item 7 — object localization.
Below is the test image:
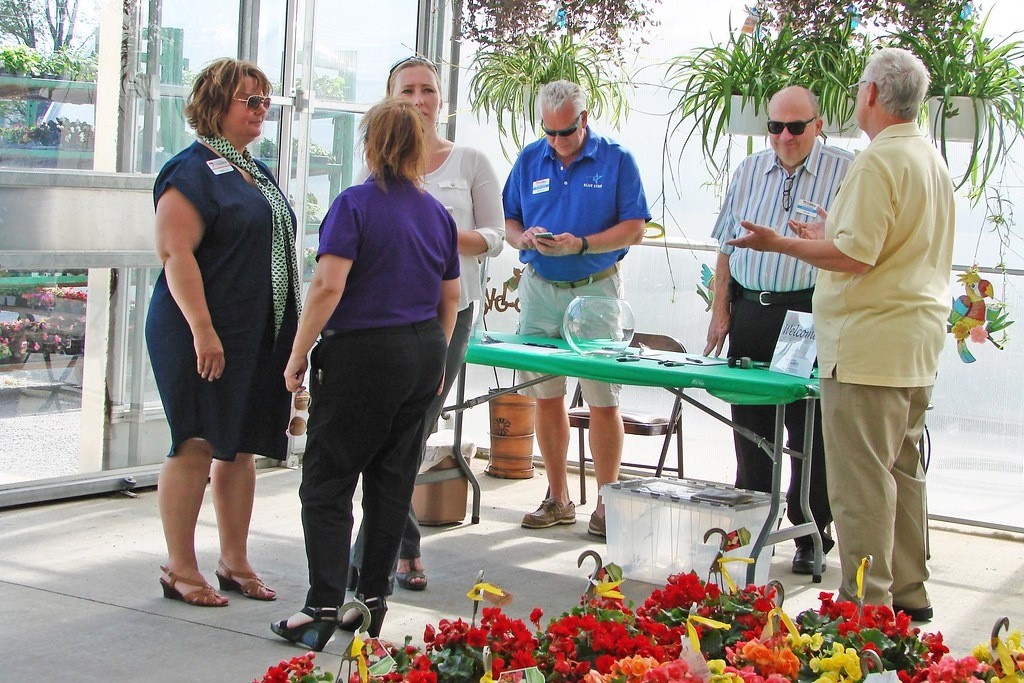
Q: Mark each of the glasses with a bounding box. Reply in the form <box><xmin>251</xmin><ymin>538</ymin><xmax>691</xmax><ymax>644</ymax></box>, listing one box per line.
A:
<box><xmin>782</xmin><ymin>175</ymin><xmax>795</xmax><ymax>213</ymax></box>
<box><xmin>232</xmin><ymin>94</ymin><xmax>271</xmax><ymax>111</ymax></box>
<box><xmin>388</xmin><ymin>55</ymin><xmax>437</xmax><ymax>78</ymax></box>
<box><xmin>540</xmin><ymin>112</ymin><xmax>583</xmax><ymax>137</ymax></box>
<box><xmin>766</xmin><ymin>115</ymin><xmax>819</xmax><ymax>136</ymax></box>
<box><xmin>848</xmin><ymin>80</ymin><xmax>869</xmax><ymax>102</ymax></box>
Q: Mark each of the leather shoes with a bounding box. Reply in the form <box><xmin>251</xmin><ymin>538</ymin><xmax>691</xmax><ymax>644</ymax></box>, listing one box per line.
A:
<box><xmin>792</xmin><ymin>545</ymin><xmax>827</xmax><ymax>573</ymax></box>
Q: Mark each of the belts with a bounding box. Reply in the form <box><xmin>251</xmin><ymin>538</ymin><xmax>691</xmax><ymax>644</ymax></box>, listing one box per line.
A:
<box><xmin>527</xmin><ymin>261</ymin><xmax>620</xmax><ymax>289</ymax></box>
<box><xmin>738</xmin><ymin>288</ymin><xmax>813</xmax><ymax>306</ymax></box>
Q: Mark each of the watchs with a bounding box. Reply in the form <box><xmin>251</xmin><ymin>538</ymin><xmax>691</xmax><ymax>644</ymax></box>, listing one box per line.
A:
<box><xmin>578</xmin><ymin>236</ymin><xmax>589</xmax><ymax>257</ymax></box>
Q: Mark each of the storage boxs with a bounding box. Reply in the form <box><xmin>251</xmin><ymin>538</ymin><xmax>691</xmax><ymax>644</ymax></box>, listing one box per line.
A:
<box><xmin>599</xmin><ymin>475</ymin><xmax>785</xmax><ymax>594</ymax></box>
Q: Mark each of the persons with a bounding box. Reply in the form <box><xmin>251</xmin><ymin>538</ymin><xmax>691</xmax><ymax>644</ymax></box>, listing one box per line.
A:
<box><xmin>380</xmin><ymin>54</ymin><xmax>503</xmax><ymax>592</ymax></box>
<box><xmin>723</xmin><ymin>48</ymin><xmax>957</xmax><ymax>619</ymax></box>
<box><xmin>503</xmin><ymin>80</ymin><xmax>652</xmax><ymax>538</ymax></box>
<box><xmin>701</xmin><ymin>84</ymin><xmax>835</xmax><ymax>574</ymax></box>
<box><xmin>144</xmin><ymin>57</ymin><xmax>303</xmax><ymax>607</ymax></box>
<box><xmin>268</xmin><ymin>97</ymin><xmax>462</xmax><ymax>653</ymax></box>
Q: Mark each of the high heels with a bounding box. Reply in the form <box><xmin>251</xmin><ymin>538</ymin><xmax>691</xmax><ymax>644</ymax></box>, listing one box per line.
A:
<box><xmin>270</xmin><ymin>607</ymin><xmax>339</xmax><ymax>651</ymax></box>
<box><xmin>337</xmin><ymin>591</ymin><xmax>388</xmax><ymax>640</ymax></box>
<box><xmin>160</xmin><ymin>564</ymin><xmax>229</xmax><ymax>607</ymax></box>
<box><xmin>214</xmin><ymin>559</ymin><xmax>276</xmax><ymax>601</ymax></box>
<box><xmin>395</xmin><ymin>556</ymin><xmax>427</xmax><ymax>590</ymax></box>
<box><xmin>346</xmin><ymin>564</ymin><xmax>394</xmax><ymax>596</ymax></box>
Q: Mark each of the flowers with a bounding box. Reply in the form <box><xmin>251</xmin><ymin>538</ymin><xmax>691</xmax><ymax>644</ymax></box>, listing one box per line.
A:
<box><xmin>0</xmin><ymin>118</ymin><xmax>94</xmax><ymax>150</ymax></box>
<box><xmin>252</xmin><ymin>570</ymin><xmax>1024</xmax><ymax>682</ymax></box>
<box><xmin>0</xmin><ymin>284</ymin><xmax>87</xmax><ymax>359</ymax></box>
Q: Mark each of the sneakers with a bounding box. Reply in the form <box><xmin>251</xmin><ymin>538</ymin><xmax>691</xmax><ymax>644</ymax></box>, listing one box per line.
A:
<box><xmin>586</xmin><ymin>510</ymin><xmax>606</xmax><ymax>535</ymax></box>
<box><xmin>521</xmin><ymin>499</ymin><xmax>577</xmax><ymax>528</ymax></box>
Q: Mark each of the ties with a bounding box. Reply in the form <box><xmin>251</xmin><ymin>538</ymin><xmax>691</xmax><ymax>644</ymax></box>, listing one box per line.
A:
<box><xmin>201</xmin><ymin>129</ymin><xmax>302</xmax><ymax>353</ymax></box>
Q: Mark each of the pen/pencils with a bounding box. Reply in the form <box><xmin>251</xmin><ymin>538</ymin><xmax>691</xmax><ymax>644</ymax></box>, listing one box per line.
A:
<box><xmin>658</xmin><ymin>360</ymin><xmax>669</xmax><ymax>364</ymax></box>
<box><xmin>523</xmin><ymin>343</ymin><xmax>558</xmax><ymax>348</ymax></box>
<box><xmin>685</xmin><ymin>358</ymin><xmax>703</xmax><ymax>363</ymax></box>
<box><xmin>616</xmin><ymin>358</ymin><xmax>640</xmax><ymax>362</ymax></box>
<box><xmin>663</xmin><ymin>363</ymin><xmax>685</xmax><ymax>366</ymax></box>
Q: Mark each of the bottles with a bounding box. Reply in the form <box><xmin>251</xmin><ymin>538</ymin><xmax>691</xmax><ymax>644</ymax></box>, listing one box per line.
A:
<box><xmin>563</xmin><ymin>295</ymin><xmax>636</xmax><ymax>357</ymax></box>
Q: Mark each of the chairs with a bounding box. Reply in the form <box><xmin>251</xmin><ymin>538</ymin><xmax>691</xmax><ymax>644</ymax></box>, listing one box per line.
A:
<box><xmin>544</xmin><ymin>333</ymin><xmax>686</xmax><ymax>505</ymax></box>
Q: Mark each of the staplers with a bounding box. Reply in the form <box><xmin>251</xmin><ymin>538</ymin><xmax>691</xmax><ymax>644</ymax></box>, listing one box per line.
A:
<box><xmin>727</xmin><ymin>356</ymin><xmax>751</xmax><ymax>369</ymax></box>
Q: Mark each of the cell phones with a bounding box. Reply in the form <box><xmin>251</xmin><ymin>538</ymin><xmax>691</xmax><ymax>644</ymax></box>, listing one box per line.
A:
<box><xmin>534</xmin><ymin>233</ymin><xmax>555</xmax><ymax>246</ymax></box>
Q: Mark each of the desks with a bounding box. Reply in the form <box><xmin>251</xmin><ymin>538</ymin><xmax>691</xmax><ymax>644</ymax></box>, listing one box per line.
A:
<box><xmin>435</xmin><ymin>332</ymin><xmax>824</xmax><ymax>589</ymax></box>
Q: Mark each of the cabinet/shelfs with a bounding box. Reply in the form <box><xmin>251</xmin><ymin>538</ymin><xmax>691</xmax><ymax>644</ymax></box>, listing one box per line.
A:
<box><xmin>0</xmin><ymin>76</ymin><xmax>382</xmax><ymax>406</ymax></box>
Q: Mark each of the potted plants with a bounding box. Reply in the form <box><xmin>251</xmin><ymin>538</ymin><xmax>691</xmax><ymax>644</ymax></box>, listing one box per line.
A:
<box><xmin>399</xmin><ymin>9</ymin><xmax>630</xmax><ymax>169</ymax></box>
<box><xmin>0</xmin><ymin>47</ymin><xmax>99</xmax><ymax>104</ymax></box>
<box><xmin>663</xmin><ymin>2</ymin><xmax>1024</xmax><ymax>344</ymax></box>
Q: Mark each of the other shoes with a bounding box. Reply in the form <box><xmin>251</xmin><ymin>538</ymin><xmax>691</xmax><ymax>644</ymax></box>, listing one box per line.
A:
<box><xmin>892</xmin><ymin>604</ymin><xmax>933</xmax><ymax>620</ymax></box>
